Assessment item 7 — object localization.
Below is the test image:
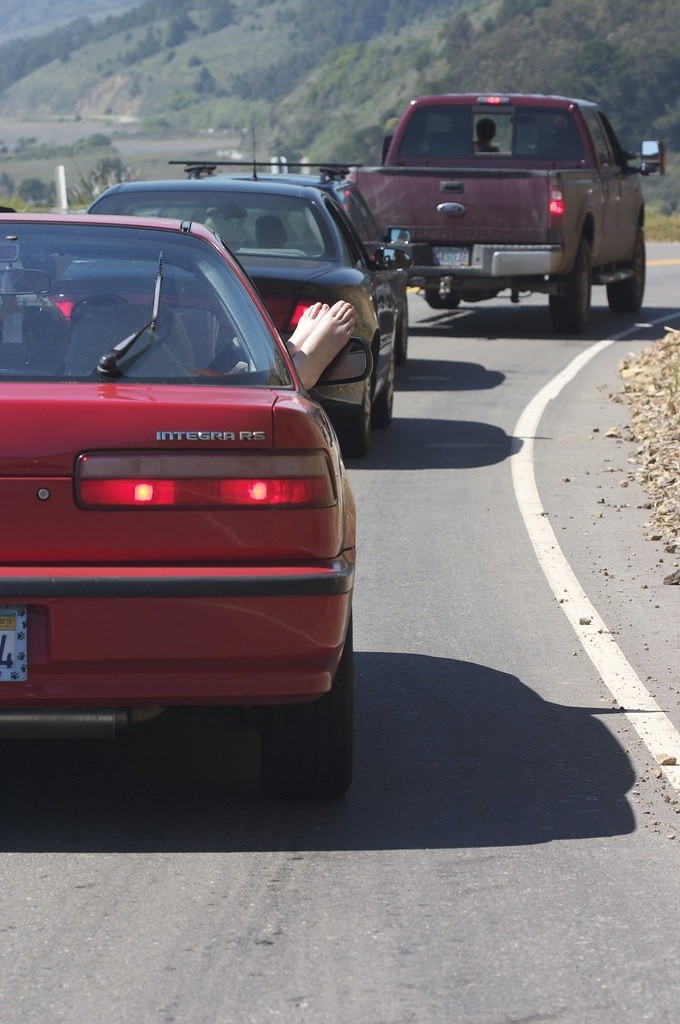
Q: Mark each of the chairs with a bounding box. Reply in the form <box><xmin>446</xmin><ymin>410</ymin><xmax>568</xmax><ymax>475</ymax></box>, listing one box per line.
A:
<box><xmin>65</xmin><ymin>306</ymin><xmax>195</xmax><ymax>380</ymax></box>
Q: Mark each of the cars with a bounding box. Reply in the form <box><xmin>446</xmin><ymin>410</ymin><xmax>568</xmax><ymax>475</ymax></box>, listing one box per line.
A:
<box><xmin>0</xmin><ymin>203</ymin><xmax>357</xmax><ymax>802</ymax></box>
<box><xmin>52</xmin><ymin>154</ymin><xmax>416</xmax><ymax>448</ymax></box>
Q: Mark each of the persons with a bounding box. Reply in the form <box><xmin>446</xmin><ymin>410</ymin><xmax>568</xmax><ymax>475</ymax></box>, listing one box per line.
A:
<box><xmin>285</xmin><ymin>300</ymin><xmax>356</xmax><ymax>390</ymax></box>
<box><xmin>476</xmin><ymin>118</ymin><xmax>499</xmax><ymax>152</ymax></box>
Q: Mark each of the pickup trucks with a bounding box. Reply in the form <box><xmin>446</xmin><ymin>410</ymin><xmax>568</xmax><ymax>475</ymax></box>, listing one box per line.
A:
<box><xmin>340</xmin><ymin>89</ymin><xmax>665</xmax><ymax>337</ymax></box>
